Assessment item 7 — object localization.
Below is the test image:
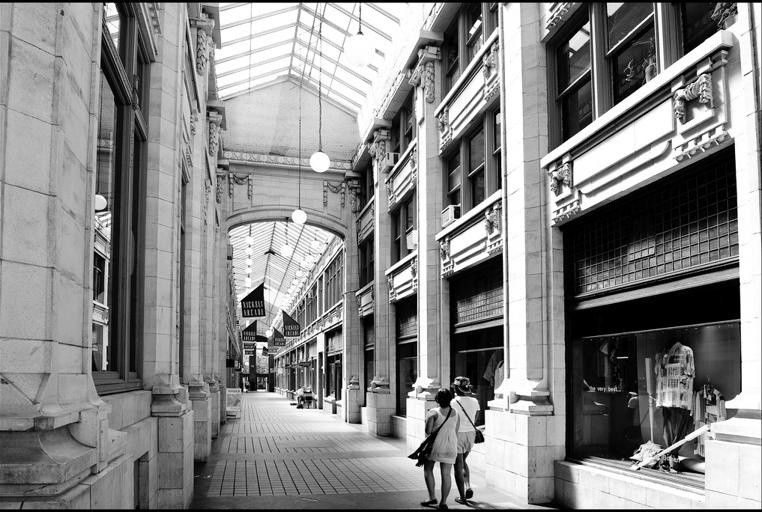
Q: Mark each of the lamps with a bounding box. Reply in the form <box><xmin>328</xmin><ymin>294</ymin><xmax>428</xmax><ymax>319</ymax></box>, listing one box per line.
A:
<box><xmin>343</xmin><ymin>2</ymin><xmax>375</xmax><ymax>68</ymax></box>
<box><xmin>280</xmin><ymin>3</ymin><xmax>330</xmax><ymax>257</ymax></box>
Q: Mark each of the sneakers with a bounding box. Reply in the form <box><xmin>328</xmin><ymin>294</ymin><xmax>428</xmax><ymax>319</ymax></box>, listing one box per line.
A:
<box><xmin>455</xmin><ymin>488</ymin><xmax>474</xmax><ymax>503</ymax></box>
<box><xmin>421</xmin><ymin>498</ymin><xmax>448</xmax><ymax>509</ymax></box>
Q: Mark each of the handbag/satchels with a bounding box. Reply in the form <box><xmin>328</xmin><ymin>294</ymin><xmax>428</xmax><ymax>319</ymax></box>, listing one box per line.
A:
<box><xmin>409</xmin><ymin>430</ymin><xmax>439</xmax><ymax>466</ymax></box>
<box><xmin>474</xmin><ymin>430</ymin><xmax>485</xmax><ymax>444</ymax></box>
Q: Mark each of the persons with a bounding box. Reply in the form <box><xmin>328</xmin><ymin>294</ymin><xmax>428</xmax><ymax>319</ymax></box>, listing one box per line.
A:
<box><xmin>654</xmin><ymin>330</ymin><xmax>696</xmax><ymax>474</ymax></box>
<box><xmin>303</xmin><ymin>386</ymin><xmax>313</xmax><ymax>409</ymax></box>
<box><xmin>295</xmin><ymin>385</ymin><xmax>304</xmax><ymax>408</ymax></box>
<box><xmin>420</xmin><ymin>388</ymin><xmax>461</xmax><ymax>510</ymax></box>
<box><xmin>449</xmin><ymin>375</ymin><xmax>481</xmax><ymax>504</ymax></box>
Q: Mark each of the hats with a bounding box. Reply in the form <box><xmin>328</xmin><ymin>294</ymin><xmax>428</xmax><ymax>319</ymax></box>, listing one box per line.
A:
<box><xmin>435</xmin><ymin>389</ymin><xmax>452</xmax><ymax>402</ymax></box>
<box><xmin>451</xmin><ymin>375</ymin><xmax>474</xmax><ymax>394</ymax></box>
